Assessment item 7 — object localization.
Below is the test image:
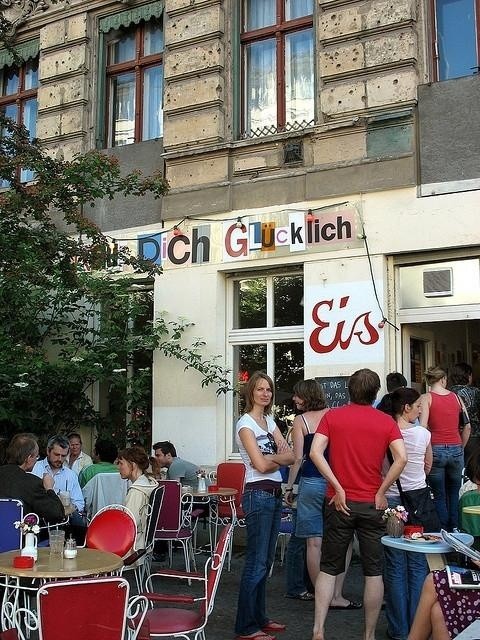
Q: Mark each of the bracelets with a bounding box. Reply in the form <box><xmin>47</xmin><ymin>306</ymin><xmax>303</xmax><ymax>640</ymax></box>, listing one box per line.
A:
<box><xmin>13</xmin><ymin>556</ymin><xmax>34</xmax><ymax>568</ymax></box>
<box><xmin>285</xmin><ymin>487</ymin><xmax>293</xmax><ymax>491</ymax></box>
<box><xmin>404</xmin><ymin>525</ymin><xmax>424</xmax><ymax>536</ymax></box>
<box><xmin>208</xmin><ymin>485</ymin><xmax>219</xmax><ymax>492</ymax></box>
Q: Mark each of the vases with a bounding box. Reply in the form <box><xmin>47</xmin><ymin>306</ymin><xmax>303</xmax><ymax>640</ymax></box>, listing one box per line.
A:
<box><xmin>379</xmin><ymin>505</ymin><xmax>409</xmax><ymax>522</ymax></box>
<box><xmin>386</xmin><ymin>516</ymin><xmax>405</xmax><ymax>538</ymax></box>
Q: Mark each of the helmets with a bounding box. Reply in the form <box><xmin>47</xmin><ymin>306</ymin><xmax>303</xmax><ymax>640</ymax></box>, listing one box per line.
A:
<box><xmin>401</xmin><ymin>535</ymin><xmax>442</xmax><ymax>544</ymax></box>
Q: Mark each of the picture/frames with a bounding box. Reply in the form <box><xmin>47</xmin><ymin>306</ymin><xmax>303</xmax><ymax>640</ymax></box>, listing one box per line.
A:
<box><xmin>379</xmin><ymin>532</ymin><xmax>475</xmax><ymax>574</ymax></box>
<box><xmin>462</xmin><ymin>505</ymin><xmax>480</xmax><ymax>516</ymax></box>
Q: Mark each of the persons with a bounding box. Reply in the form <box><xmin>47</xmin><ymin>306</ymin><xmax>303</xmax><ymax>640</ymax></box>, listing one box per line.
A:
<box><xmin>382</xmin><ymin>389</ymin><xmax>433</xmax><ymax>638</ymax></box>
<box><xmin>0</xmin><ymin>419</ymin><xmax>159</xmax><ymax>594</ymax></box>
<box><xmin>446</xmin><ymin>366</ymin><xmax>480</xmax><ymax>468</ymax></box>
<box><xmin>408</xmin><ymin>449</ymin><xmax>480</xmax><ymax>639</ymax></box>
<box><xmin>309</xmin><ymin>369</ymin><xmax>408</xmax><ymax>638</ymax></box>
<box><xmin>234</xmin><ymin>370</ymin><xmax>295</xmax><ymax>638</ymax></box>
<box><xmin>417</xmin><ymin>365</ymin><xmax>471</xmax><ymax>533</ymax></box>
<box><xmin>152</xmin><ymin>441</ymin><xmax>205</xmax><ymax>563</ymax></box>
<box><xmin>283</xmin><ymin>380</ymin><xmax>363</xmax><ymax>610</ymax></box>
<box><xmin>376</xmin><ymin>372</ymin><xmax>419</xmax><ymax>605</ymax></box>
<box><xmin>284</xmin><ymin>499</ymin><xmax>314</xmax><ymax>601</ymax></box>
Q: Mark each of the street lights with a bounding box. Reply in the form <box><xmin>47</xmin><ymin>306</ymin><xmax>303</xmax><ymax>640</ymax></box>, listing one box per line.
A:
<box><xmin>460</xmin><ymin>411</ymin><xmax>469</xmax><ymax>426</ymax></box>
<box><xmin>403</xmin><ymin>488</ymin><xmax>441</xmax><ymax>533</ymax></box>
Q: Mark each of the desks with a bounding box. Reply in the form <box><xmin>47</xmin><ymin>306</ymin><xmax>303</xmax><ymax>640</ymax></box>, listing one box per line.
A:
<box><xmin>462</xmin><ymin>505</ymin><xmax>480</xmax><ymax>516</ymax></box>
<box><xmin>379</xmin><ymin>532</ymin><xmax>475</xmax><ymax>574</ymax></box>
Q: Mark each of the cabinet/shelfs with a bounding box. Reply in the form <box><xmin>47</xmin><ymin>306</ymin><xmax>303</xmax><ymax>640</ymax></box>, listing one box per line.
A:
<box><xmin>63</xmin><ymin>560</ymin><xmax>78</xmax><ymax>571</ymax></box>
<box><xmin>63</xmin><ymin>539</ymin><xmax>79</xmax><ymax>560</ymax></box>
<box><xmin>197</xmin><ymin>476</ymin><xmax>206</xmax><ymax>493</ymax></box>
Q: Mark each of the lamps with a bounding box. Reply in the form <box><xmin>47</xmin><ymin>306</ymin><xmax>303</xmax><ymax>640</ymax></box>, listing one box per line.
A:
<box><xmin>270</xmin><ymin>464</ymin><xmax>301</xmax><ymax>578</ymax></box>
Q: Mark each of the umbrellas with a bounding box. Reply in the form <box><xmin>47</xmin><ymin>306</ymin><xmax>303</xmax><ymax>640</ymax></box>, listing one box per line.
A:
<box><xmin>296</xmin><ymin>592</ymin><xmax>314</xmax><ymax>600</ymax></box>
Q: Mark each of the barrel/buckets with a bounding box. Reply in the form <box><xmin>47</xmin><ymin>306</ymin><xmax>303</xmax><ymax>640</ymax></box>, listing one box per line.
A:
<box><xmin>236</xmin><ymin>634</ymin><xmax>276</xmax><ymax>640</ymax></box>
<box><xmin>262</xmin><ymin>620</ymin><xmax>286</xmax><ymax>631</ymax></box>
<box><xmin>329</xmin><ymin>599</ymin><xmax>362</xmax><ymax>610</ymax></box>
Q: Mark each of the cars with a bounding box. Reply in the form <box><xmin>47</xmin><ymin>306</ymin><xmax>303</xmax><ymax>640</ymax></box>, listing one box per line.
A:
<box><xmin>48</xmin><ymin>553</ymin><xmax>63</xmax><ymax>573</ymax></box>
<box><xmin>59</xmin><ymin>491</ymin><xmax>71</xmax><ymax>508</ymax></box>
<box><xmin>49</xmin><ymin>529</ymin><xmax>65</xmax><ymax>554</ymax></box>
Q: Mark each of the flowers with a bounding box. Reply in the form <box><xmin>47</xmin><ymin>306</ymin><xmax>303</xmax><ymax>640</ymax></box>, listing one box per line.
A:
<box><xmin>379</xmin><ymin>505</ymin><xmax>409</xmax><ymax>522</ymax></box>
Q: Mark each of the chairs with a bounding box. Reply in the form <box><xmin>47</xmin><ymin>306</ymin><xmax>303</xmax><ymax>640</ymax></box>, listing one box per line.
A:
<box><xmin>0</xmin><ymin>461</ymin><xmax>246</xmax><ymax>640</ymax></box>
<box><xmin>270</xmin><ymin>464</ymin><xmax>301</xmax><ymax>578</ymax></box>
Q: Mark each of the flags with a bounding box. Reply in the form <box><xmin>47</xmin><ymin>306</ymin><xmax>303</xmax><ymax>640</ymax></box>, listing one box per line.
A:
<box><xmin>386</xmin><ymin>516</ymin><xmax>405</xmax><ymax>538</ymax></box>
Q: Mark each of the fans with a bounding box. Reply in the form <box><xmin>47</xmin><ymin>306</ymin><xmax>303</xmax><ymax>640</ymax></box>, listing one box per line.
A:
<box><xmin>315</xmin><ymin>376</ymin><xmax>351</xmax><ymax>409</ymax></box>
